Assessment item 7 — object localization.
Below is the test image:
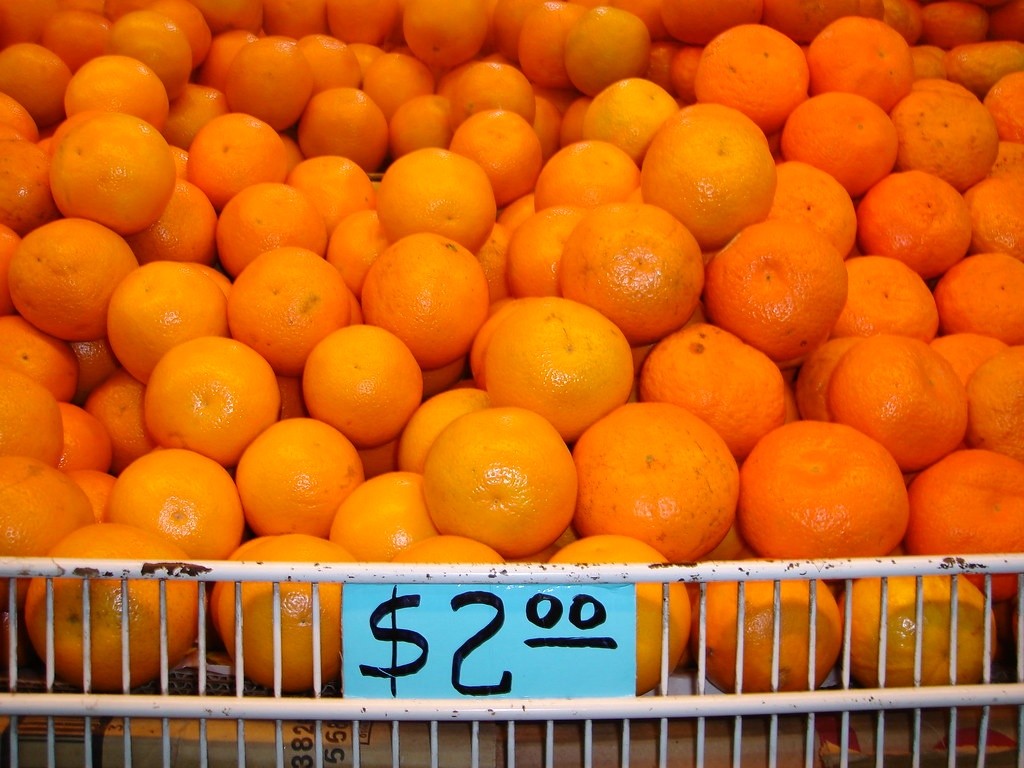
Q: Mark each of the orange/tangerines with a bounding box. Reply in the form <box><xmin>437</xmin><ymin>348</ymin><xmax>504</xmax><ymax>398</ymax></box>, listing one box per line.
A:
<box><xmin>0</xmin><ymin>0</ymin><xmax>1024</xmax><ymax>691</ymax></box>
